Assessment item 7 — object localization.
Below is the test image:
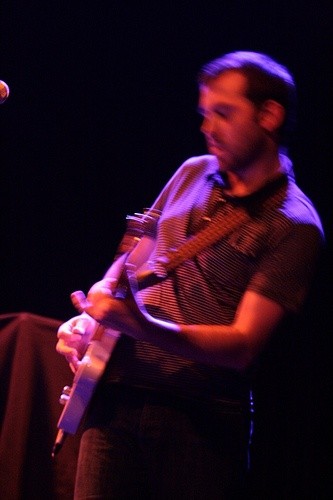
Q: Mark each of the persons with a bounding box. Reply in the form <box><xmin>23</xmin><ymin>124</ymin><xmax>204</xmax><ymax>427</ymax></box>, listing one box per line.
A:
<box><xmin>52</xmin><ymin>51</ymin><xmax>328</xmax><ymax>500</ymax></box>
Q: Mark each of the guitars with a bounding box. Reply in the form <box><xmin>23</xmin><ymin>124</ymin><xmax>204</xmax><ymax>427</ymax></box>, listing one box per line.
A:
<box><xmin>57</xmin><ymin>206</ymin><xmax>165</xmax><ymax>435</ymax></box>
